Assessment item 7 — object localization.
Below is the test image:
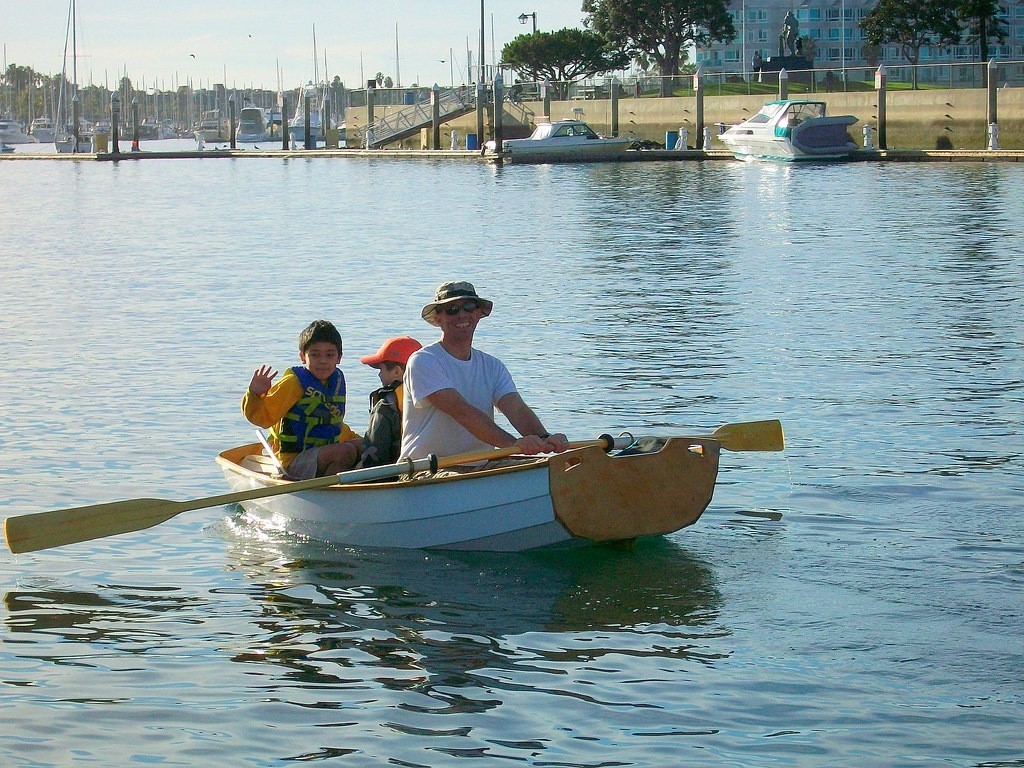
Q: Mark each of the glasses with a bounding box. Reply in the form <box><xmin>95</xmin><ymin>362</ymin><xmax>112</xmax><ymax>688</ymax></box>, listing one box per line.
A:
<box><xmin>438</xmin><ymin>301</ymin><xmax>477</xmax><ymax>315</ymax></box>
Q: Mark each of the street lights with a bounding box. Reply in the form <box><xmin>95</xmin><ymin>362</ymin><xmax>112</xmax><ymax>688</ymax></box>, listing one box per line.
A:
<box><xmin>518</xmin><ymin>12</ymin><xmax>538</xmax><ymax>90</ymax></box>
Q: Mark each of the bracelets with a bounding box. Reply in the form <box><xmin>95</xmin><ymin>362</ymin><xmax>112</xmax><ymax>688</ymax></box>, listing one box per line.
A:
<box><xmin>539</xmin><ymin>433</ymin><xmax>551</xmax><ymax>438</ymax></box>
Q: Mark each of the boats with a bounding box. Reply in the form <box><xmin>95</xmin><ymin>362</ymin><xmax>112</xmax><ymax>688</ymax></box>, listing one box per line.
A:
<box><xmin>0</xmin><ymin>142</ymin><xmax>16</xmax><ymax>153</ymax></box>
<box><xmin>717</xmin><ymin>99</ymin><xmax>859</xmax><ymax>163</ymax></box>
<box><xmin>214</xmin><ymin>431</ymin><xmax>721</xmax><ymax>558</ymax></box>
<box><xmin>479</xmin><ymin>118</ymin><xmax>636</xmax><ymax>164</ymax></box>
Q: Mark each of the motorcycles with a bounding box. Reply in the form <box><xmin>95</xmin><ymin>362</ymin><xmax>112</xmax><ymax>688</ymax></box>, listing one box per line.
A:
<box><xmin>502</xmin><ymin>89</ymin><xmax>521</xmax><ymax>103</ymax></box>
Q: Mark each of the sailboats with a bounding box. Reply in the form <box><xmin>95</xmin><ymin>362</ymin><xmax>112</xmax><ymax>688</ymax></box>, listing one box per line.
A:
<box><xmin>0</xmin><ymin>22</ymin><xmax>404</xmax><ymax>143</ymax></box>
<box><xmin>53</xmin><ymin>0</ymin><xmax>94</xmax><ymax>153</ymax></box>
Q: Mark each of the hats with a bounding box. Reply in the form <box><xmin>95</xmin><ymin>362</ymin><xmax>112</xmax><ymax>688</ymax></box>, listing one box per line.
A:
<box><xmin>421</xmin><ymin>280</ymin><xmax>493</xmax><ymax>327</ymax></box>
<box><xmin>361</xmin><ymin>335</ymin><xmax>422</xmax><ymax>368</ymax></box>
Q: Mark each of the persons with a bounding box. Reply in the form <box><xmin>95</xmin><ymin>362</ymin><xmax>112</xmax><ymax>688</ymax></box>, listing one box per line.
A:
<box><xmin>783</xmin><ymin>11</ymin><xmax>799</xmax><ymax>57</ymax></box>
<box><xmin>825</xmin><ymin>71</ymin><xmax>833</xmax><ymax>93</ymax></box>
<box><xmin>242</xmin><ymin>320</ymin><xmax>365</xmax><ymax>480</ymax></box>
<box><xmin>752</xmin><ymin>51</ymin><xmax>762</xmax><ymax>82</ymax></box>
<box><xmin>461</xmin><ymin>82</ymin><xmax>476</xmax><ymax>104</ymax></box>
<box><xmin>354</xmin><ymin>336</ymin><xmax>423</xmax><ymax>483</ymax></box>
<box><xmin>512</xmin><ymin>79</ymin><xmax>523</xmax><ymax>102</ymax></box>
<box><xmin>634</xmin><ymin>82</ymin><xmax>642</xmax><ymax>99</ymax></box>
<box><xmin>396</xmin><ymin>280</ymin><xmax>569</xmax><ymax>481</ymax></box>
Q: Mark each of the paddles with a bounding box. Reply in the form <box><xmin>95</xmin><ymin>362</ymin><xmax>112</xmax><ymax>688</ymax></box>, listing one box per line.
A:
<box><xmin>4</xmin><ymin>420</ymin><xmax>785</xmax><ymax>555</ymax></box>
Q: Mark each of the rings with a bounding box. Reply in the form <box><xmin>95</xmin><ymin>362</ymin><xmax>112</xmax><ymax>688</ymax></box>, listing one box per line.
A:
<box><xmin>562</xmin><ymin>441</ymin><xmax>567</xmax><ymax>444</ymax></box>
